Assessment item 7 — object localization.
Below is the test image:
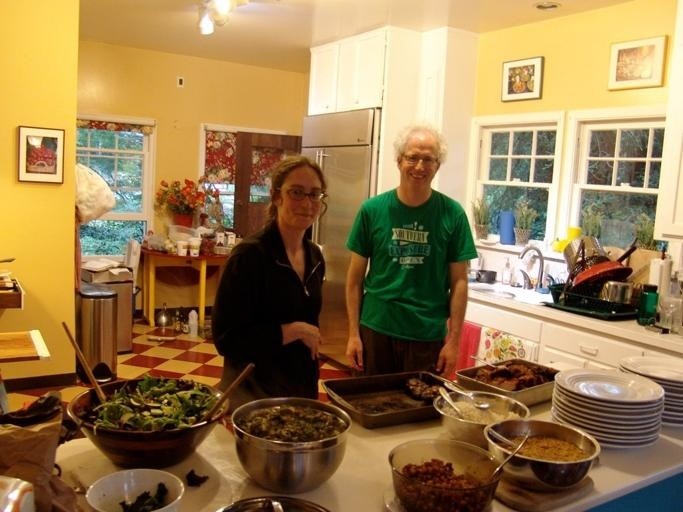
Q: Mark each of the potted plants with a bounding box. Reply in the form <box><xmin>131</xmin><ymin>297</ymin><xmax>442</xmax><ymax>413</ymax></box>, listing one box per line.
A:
<box><xmin>512</xmin><ymin>193</ymin><xmax>537</xmax><ymax>247</ymax></box>
<box><xmin>470</xmin><ymin>197</ymin><xmax>490</xmax><ymax>240</ymax></box>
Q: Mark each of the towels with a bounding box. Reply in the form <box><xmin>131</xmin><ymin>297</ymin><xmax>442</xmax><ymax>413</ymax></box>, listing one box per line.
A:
<box><xmin>442</xmin><ymin>319</ymin><xmax>481</xmax><ymax>382</ymax></box>
<box><xmin>475</xmin><ymin>328</ymin><xmax>538</xmax><ymax>371</ymax></box>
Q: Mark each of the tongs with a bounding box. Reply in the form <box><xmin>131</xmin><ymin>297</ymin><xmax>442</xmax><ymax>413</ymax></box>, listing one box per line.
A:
<box><xmin>558</xmin><ymin>240</ymin><xmax>585</xmax><ymax>306</ymax></box>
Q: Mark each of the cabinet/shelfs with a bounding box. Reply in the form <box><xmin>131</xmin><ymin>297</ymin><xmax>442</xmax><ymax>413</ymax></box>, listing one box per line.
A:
<box><xmin>538</xmin><ymin>318</ymin><xmax>646</xmax><ymax>380</ymax></box>
<box><xmin>0</xmin><ymin>275</ymin><xmax>24</xmax><ymax>318</ymax></box>
<box><xmin>81</xmin><ymin>269</ymin><xmax>133</xmax><ymax>355</ymax></box>
<box><xmin>308</xmin><ymin>25</ymin><xmax>422</xmax><ymax>116</ymax></box>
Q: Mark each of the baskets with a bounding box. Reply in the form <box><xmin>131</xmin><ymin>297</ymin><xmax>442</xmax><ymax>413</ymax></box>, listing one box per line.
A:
<box><xmin>168</xmin><ymin>224</ymin><xmax>201</xmax><ymax>243</ymax></box>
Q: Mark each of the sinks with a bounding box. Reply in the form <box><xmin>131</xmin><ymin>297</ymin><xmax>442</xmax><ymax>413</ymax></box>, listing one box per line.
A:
<box><xmin>470</xmin><ymin>285</ymin><xmax>518</xmax><ymax>303</ymax></box>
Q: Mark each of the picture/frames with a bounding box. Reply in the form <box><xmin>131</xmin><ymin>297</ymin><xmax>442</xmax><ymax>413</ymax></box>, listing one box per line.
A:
<box><xmin>501</xmin><ymin>57</ymin><xmax>544</xmax><ymax>102</ymax></box>
<box><xmin>17</xmin><ymin>126</ymin><xmax>65</xmax><ymax>184</ymax></box>
<box><xmin>609</xmin><ymin>36</ymin><xmax>668</xmax><ymax>91</ymax></box>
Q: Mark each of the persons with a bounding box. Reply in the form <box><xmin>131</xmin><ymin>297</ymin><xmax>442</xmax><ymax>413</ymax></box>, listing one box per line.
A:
<box><xmin>207</xmin><ymin>153</ymin><xmax>325</xmax><ymax>399</ymax></box>
<box><xmin>341</xmin><ymin>122</ymin><xmax>480</xmax><ymax>387</ymax></box>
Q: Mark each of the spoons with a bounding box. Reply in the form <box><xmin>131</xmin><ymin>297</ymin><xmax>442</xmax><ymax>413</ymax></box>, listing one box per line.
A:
<box><xmin>445</xmin><ymin>382</ymin><xmax>490</xmax><ymax>408</ymax></box>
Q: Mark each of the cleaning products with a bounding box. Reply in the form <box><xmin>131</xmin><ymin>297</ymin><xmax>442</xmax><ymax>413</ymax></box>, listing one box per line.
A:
<box><xmin>502</xmin><ymin>257</ymin><xmax>513</xmax><ymax>284</ymax></box>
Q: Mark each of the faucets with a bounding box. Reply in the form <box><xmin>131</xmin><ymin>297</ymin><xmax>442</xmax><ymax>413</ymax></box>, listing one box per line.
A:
<box><xmin>519</xmin><ymin>243</ymin><xmax>544</xmax><ymax>290</ymax></box>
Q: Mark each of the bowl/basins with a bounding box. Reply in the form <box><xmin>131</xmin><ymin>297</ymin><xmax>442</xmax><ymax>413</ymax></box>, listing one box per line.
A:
<box><xmin>66</xmin><ymin>377</ymin><xmax>230</xmax><ymax>468</ymax></box>
<box><xmin>86</xmin><ymin>469</ymin><xmax>184</xmax><ymax>512</ymax></box>
<box><xmin>388</xmin><ymin>439</ymin><xmax>504</xmax><ymax>512</ymax></box>
<box><xmin>231</xmin><ymin>397</ymin><xmax>352</xmax><ymax>494</ymax></box>
<box><xmin>564</xmin><ymin>227</ymin><xmax>637</xmax><ymax>305</ymax></box>
<box><xmin>484</xmin><ymin>419</ymin><xmax>601</xmax><ymax>492</ymax></box>
<box><xmin>434</xmin><ymin>391</ymin><xmax>531</xmax><ymax>450</ymax></box>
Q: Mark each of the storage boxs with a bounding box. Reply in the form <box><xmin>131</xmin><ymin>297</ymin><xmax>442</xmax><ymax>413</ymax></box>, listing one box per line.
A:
<box><xmin>168</xmin><ymin>225</ymin><xmax>202</xmax><ymax>245</ymax></box>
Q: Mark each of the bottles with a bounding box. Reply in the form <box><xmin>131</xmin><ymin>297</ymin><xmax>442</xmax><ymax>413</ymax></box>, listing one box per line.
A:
<box><xmin>174</xmin><ymin>306</ymin><xmax>198</xmax><ymax>336</ymax></box>
<box><xmin>502</xmin><ymin>257</ymin><xmax>521</xmax><ymax>287</ymax></box>
<box><xmin>500</xmin><ymin>211</ymin><xmax>515</xmax><ymax>244</ymax></box>
<box><xmin>637</xmin><ymin>243</ymin><xmax>683</xmax><ymax>336</ymax></box>
<box><xmin>157</xmin><ymin>303</ymin><xmax>171</xmax><ymax>326</ymax></box>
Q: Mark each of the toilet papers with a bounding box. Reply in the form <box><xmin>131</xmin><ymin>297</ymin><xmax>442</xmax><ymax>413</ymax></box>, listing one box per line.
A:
<box><xmin>648</xmin><ymin>256</ymin><xmax>666</xmax><ymax>294</ymax></box>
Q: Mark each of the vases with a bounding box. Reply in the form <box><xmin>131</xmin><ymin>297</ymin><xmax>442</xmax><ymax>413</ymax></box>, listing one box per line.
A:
<box><xmin>497</xmin><ymin>211</ymin><xmax>516</xmax><ymax>245</ymax></box>
<box><xmin>173</xmin><ymin>214</ymin><xmax>193</xmax><ymax>229</ymax></box>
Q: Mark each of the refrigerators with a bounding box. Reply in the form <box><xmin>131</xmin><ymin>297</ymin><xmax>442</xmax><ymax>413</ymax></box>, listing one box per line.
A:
<box><xmin>297</xmin><ymin>108</ymin><xmax>381</xmax><ymax>371</ymax></box>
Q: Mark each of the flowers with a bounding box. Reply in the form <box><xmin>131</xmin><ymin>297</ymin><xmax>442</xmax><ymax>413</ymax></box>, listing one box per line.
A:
<box><xmin>153</xmin><ymin>176</ymin><xmax>221</xmax><ymax>215</ymax></box>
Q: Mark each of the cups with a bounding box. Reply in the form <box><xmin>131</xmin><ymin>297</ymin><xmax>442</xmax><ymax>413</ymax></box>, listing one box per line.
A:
<box><xmin>188</xmin><ymin>238</ymin><xmax>202</xmax><ymax>257</ymax></box>
<box><xmin>177</xmin><ymin>241</ymin><xmax>188</xmax><ymax>256</ymax></box>
<box><xmin>476</xmin><ymin>270</ymin><xmax>497</xmax><ymax>283</ymax></box>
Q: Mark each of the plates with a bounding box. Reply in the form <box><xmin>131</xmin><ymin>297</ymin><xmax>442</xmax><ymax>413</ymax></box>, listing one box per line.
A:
<box><xmin>383</xmin><ymin>483</ymin><xmax>499</xmax><ymax>512</ymax></box>
<box><xmin>216</xmin><ymin>496</ymin><xmax>334</xmax><ymax>512</ymax></box>
<box><xmin>550</xmin><ymin>357</ymin><xmax>683</xmax><ymax>449</ymax></box>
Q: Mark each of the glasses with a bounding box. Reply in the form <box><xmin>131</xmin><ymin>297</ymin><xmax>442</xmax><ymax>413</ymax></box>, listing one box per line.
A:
<box><xmin>400</xmin><ymin>154</ymin><xmax>441</xmax><ymax>166</ymax></box>
<box><xmin>273</xmin><ymin>185</ymin><xmax>326</xmax><ymax>202</ymax></box>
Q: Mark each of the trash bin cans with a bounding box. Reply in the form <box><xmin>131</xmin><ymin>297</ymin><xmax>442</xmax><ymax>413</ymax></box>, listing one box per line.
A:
<box><xmin>77</xmin><ymin>283</ymin><xmax>117</xmax><ymax>385</ymax></box>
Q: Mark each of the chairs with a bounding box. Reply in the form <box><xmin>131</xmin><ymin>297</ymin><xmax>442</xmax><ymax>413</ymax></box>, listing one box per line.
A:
<box><xmin>119</xmin><ymin>239</ymin><xmax>140</xmax><ymax>316</ymax></box>
<box><xmin>0</xmin><ymin>369</ymin><xmax>39</xmax><ymax>416</ymax></box>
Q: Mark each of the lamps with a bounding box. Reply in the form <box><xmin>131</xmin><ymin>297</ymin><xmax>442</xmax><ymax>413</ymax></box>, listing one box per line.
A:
<box><xmin>197</xmin><ymin>0</ymin><xmax>247</xmax><ymax>35</ymax></box>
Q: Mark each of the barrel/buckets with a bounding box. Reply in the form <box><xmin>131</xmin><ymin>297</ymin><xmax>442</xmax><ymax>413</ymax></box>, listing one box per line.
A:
<box><xmin>188</xmin><ymin>309</ymin><xmax>199</xmax><ymax>336</ymax></box>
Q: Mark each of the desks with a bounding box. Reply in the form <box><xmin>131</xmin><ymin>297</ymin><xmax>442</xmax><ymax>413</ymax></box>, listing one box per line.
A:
<box><xmin>0</xmin><ymin>330</ymin><xmax>50</xmax><ymax>363</ymax></box>
<box><xmin>50</xmin><ymin>384</ymin><xmax>683</xmax><ymax>512</ymax></box>
<box><xmin>139</xmin><ymin>247</ymin><xmax>234</xmax><ymax>326</ymax></box>
